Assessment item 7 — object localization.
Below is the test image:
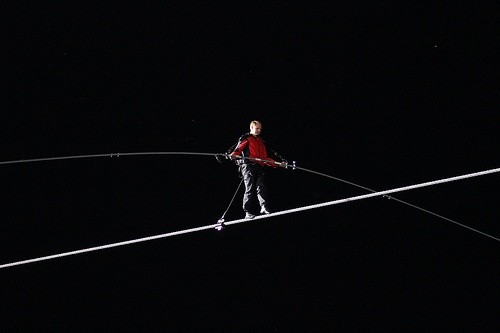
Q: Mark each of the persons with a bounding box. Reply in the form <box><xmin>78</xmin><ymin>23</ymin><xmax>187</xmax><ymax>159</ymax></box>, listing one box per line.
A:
<box><xmin>226</xmin><ymin>121</ymin><xmax>288</xmax><ymax>219</ymax></box>
